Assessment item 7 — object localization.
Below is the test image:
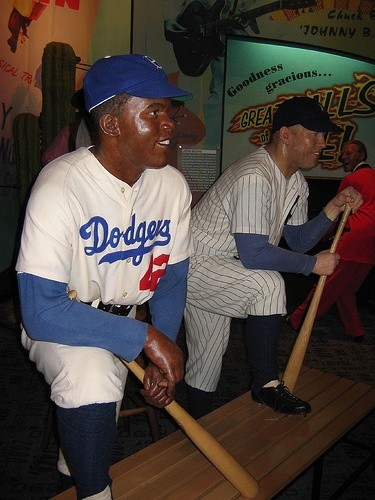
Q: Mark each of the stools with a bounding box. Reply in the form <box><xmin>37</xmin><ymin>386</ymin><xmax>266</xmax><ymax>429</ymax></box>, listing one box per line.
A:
<box><xmin>40</xmin><ymin>305</ymin><xmax>160</xmax><ymax>450</ymax></box>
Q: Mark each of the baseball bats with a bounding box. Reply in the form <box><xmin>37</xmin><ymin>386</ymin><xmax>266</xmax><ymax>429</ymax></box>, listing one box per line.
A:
<box><xmin>282</xmin><ymin>194</ymin><xmax>352</xmax><ymax>394</ymax></box>
<box><xmin>120</xmin><ymin>358</ymin><xmax>261</xmax><ymax>497</ymax></box>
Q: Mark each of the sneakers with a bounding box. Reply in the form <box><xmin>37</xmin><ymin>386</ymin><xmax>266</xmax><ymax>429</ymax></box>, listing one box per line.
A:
<box><xmin>354</xmin><ymin>335</ymin><xmax>363</xmax><ymax>343</ymax></box>
<box><xmin>251</xmin><ymin>380</ymin><xmax>311</xmax><ymax>416</ymax></box>
<box><xmin>279</xmin><ymin>320</ymin><xmax>296</xmax><ymax>332</ymax></box>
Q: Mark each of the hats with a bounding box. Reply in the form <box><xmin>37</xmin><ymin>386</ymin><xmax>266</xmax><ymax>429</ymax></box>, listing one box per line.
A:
<box><xmin>82</xmin><ymin>54</ymin><xmax>192</xmax><ymax>114</ymax></box>
<box><xmin>273</xmin><ymin>96</ymin><xmax>344</xmax><ymax>133</ymax></box>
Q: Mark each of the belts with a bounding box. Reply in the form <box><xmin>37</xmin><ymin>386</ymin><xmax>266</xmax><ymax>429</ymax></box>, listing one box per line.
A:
<box><xmin>81</xmin><ymin>301</ymin><xmax>133</xmax><ymax>316</ymax></box>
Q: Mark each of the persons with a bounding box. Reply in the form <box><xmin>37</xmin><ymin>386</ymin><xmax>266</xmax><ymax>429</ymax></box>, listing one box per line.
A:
<box><xmin>184</xmin><ymin>97</ymin><xmax>364</xmax><ymax>415</ymax></box>
<box><xmin>15</xmin><ymin>54</ymin><xmax>193</xmax><ymax>500</ymax></box>
<box><xmin>285</xmin><ymin>140</ymin><xmax>375</xmax><ymax>341</ymax></box>
<box><xmin>40</xmin><ymin>88</ymin><xmax>93</xmax><ymax>165</ymax></box>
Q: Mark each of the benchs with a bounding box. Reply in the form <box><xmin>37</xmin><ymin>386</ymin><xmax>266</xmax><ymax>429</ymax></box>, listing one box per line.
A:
<box><xmin>48</xmin><ymin>365</ymin><xmax>375</xmax><ymax>500</ymax></box>
